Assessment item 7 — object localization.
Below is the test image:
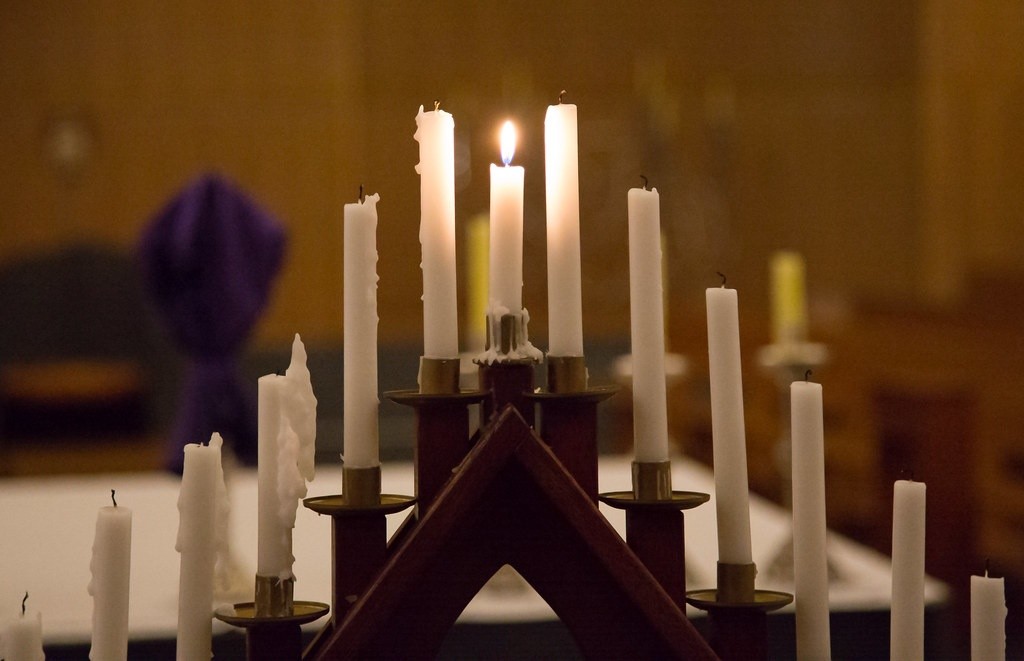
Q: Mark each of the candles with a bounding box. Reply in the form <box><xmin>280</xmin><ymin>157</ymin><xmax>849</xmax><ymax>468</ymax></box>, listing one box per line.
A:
<box><xmin>773</xmin><ymin>253</ymin><xmax>807</xmax><ymax>346</ymax></box>
<box><xmin>467</xmin><ymin>213</ymin><xmax>490</xmax><ymax>336</ymax></box>
<box><xmin>789</xmin><ymin>380</ymin><xmax>831</xmax><ymax>661</ymax></box>
<box><xmin>258</xmin><ymin>334</ymin><xmax>319</xmax><ymax>582</ymax></box>
<box><xmin>628</xmin><ymin>188</ymin><xmax>668</xmax><ymax>461</ymax></box>
<box><xmin>890</xmin><ymin>479</ymin><xmax>925</xmax><ymax>661</ymax></box>
<box><xmin>706</xmin><ymin>287</ymin><xmax>753</xmax><ymax>564</ymax></box>
<box><xmin>969</xmin><ymin>575</ymin><xmax>1008</xmax><ymax>661</ymax></box>
<box><xmin>175</xmin><ymin>432</ymin><xmax>224</xmax><ymax>661</ymax></box>
<box><xmin>489</xmin><ymin>163</ymin><xmax>524</xmax><ymax>313</ymax></box>
<box><xmin>2</xmin><ymin>610</ymin><xmax>45</xmax><ymax>661</ymax></box>
<box><xmin>413</xmin><ymin>101</ymin><xmax>457</xmax><ymax>358</ymax></box>
<box><xmin>544</xmin><ymin>103</ymin><xmax>584</xmax><ymax>355</ymax></box>
<box><xmin>342</xmin><ymin>193</ymin><xmax>383</xmax><ymax>468</ymax></box>
<box><xmin>86</xmin><ymin>506</ymin><xmax>133</xmax><ymax>661</ymax></box>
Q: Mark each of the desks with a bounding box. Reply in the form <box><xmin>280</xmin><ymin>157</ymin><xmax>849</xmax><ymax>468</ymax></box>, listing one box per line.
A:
<box><xmin>0</xmin><ymin>451</ymin><xmax>950</xmax><ymax>661</ymax></box>
